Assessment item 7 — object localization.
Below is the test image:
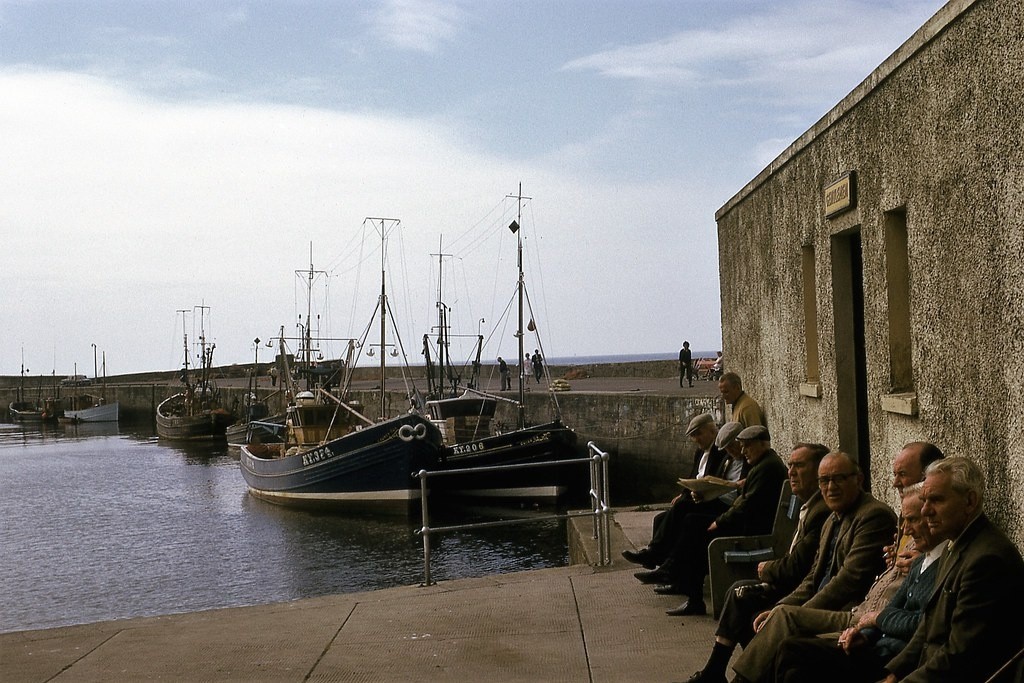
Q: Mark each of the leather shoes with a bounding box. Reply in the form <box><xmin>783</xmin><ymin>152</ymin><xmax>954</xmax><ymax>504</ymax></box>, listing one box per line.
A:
<box><xmin>622</xmin><ymin>547</ymin><xmax>656</xmax><ymax>570</ymax></box>
<box><xmin>665</xmin><ymin>601</ymin><xmax>706</xmax><ymax>615</ymax></box>
<box><xmin>634</xmin><ymin>564</ymin><xmax>673</xmax><ymax>585</ymax></box>
<box><xmin>654</xmin><ymin>584</ymin><xmax>684</xmax><ymax>594</ymax></box>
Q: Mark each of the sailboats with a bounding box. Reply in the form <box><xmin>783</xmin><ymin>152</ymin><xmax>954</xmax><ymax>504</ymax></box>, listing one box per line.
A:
<box><xmin>8</xmin><ymin>345</ymin><xmax>47</xmax><ymax>424</ymax></box>
<box><xmin>237</xmin><ymin>217</ymin><xmax>442</xmax><ymax>517</ymax></box>
<box><xmin>405</xmin><ymin>251</ymin><xmax>500</xmax><ymax>429</ymax></box>
<box><xmin>264</xmin><ymin>239</ymin><xmax>365</xmax><ymax>445</ymax></box>
<box><xmin>412</xmin><ymin>182</ymin><xmax>585</xmax><ymax>510</ymax></box>
<box><xmin>61</xmin><ymin>344</ymin><xmax>120</xmax><ymax>422</ymax></box>
<box><xmin>46</xmin><ymin>369</ymin><xmax>63</xmax><ymax>413</ymax></box>
<box><xmin>155</xmin><ymin>298</ymin><xmax>236</xmax><ymax>442</ymax></box>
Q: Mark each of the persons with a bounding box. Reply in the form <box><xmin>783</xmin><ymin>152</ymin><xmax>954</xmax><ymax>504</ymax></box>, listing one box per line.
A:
<box><xmin>718</xmin><ymin>372</ymin><xmax>763</xmax><ymax>428</ymax></box>
<box><xmin>506</xmin><ymin>367</ymin><xmax>512</xmax><ymax>390</ymax></box>
<box><xmin>679</xmin><ymin>341</ymin><xmax>695</xmax><ymax>388</ymax></box>
<box><xmin>270</xmin><ymin>364</ymin><xmax>277</xmax><ymax>386</ymax></box>
<box><xmin>617</xmin><ymin>410</ymin><xmax>956</xmax><ymax>683</ymax></box>
<box><xmin>876</xmin><ymin>456</ymin><xmax>1024</xmax><ymax>683</ymax></box>
<box><xmin>523</xmin><ymin>353</ymin><xmax>533</xmax><ymax>384</ymax></box>
<box><xmin>532</xmin><ymin>349</ymin><xmax>544</xmax><ymax>384</ymax></box>
<box><xmin>250</xmin><ymin>396</ymin><xmax>261</xmax><ymax>421</ymax></box>
<box><xmin>702</xmin><ymin>351</ymin><xmax>723</xmax><ymax>381</ymax></box>
<box><xmin>310</xmin><ymin>360</ymin><xmax>340</xmax><ymax>389</ymax></box>
<box><xmin>497</xmin><ymin>356</ymin><xmax>507</xmax><ymax>391</ymax></box>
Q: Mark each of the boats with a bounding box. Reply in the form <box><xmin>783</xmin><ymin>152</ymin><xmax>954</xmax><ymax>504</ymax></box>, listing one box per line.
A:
<box><xmin>226</xmin><ymin>337</ymin><xmax>291</xmax><ymax>448</ymax></box>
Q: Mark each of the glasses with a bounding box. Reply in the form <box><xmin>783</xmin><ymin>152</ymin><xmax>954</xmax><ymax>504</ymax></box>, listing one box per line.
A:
<box><xmin>817</xmin><ymin>472</ymin><xmax>857</xmax><ymax>485</ymax></box>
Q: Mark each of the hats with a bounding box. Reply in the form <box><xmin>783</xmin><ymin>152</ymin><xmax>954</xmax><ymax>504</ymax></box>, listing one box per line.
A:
<box><xmin>735</xmin><ymin>425</ymin><xmax>769</xmax><ymax>441</ymax></box>
<box><xmin>683</xmin><ymin>341</ymin><xmax>689</xmax><ymax>347</ymax></box>
<box><xmin>715</xmin><ymin>421</ymin><xmax>743</xmax><ymax>450</ymax></box>
<box><xmin>685</xmin><ymin>414</ymin><xmax>712</xmax><ymax>437</ymax></box>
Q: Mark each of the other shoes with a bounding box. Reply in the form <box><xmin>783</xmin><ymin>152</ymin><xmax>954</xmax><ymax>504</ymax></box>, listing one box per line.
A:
<box><xmin>671</xmin><ymin>671</ymin><xmax>728</xmax><ymax>683</ymax></box>
<box><xmin>689</xmin><ymin>384</ymin><xmax>694</xmax><ymax>387</ymax></box>
<box><xmin>680</xmin><ymin>384</ymin><xmax>683</xmax><ymax>387</ymax></box>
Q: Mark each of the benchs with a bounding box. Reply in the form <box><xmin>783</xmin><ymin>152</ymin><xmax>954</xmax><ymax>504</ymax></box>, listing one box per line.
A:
<box><xmin>694</xmin><ymin>358</ymin><xmax>723</xmax><ymax>381</ymax></box>
<box><xmin>708</xmin><ymin>478</ymin><xmax>802</xmax><ymax>619</ymax></box>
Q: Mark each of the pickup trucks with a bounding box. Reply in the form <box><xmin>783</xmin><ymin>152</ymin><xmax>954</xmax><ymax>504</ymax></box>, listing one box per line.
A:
<box><xmin>61</xmin><ymin>375</ymin><xmax>91</xmax><ymax>387</ymax></box>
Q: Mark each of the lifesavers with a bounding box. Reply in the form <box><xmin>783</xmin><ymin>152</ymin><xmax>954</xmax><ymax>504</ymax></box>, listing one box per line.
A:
<box><xmin>398</xmin><ymin>423</ymin><xmax>427</xmax><ymax>442</ymax></box>
